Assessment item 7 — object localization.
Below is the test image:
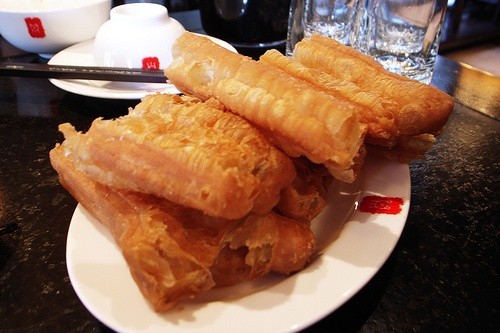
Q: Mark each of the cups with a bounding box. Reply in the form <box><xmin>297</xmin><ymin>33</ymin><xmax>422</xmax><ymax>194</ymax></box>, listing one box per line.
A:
<box><xmin>286</xmin><ymin>0</ymin><xmax>449</xmax><ymax>85</ymax></box>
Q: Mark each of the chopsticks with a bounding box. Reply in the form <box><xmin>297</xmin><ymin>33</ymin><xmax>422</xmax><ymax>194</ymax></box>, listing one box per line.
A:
<box><xmin>0</xmin><ymin>62</ymin><xmax>168</xmax><ymax>83</ymax></box>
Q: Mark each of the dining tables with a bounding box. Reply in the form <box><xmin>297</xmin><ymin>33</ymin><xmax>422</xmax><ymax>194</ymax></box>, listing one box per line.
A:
<box><xmin>0</xmin><ymin>29</ymin><xmax>500</xmax><ymax>333</ymax></box>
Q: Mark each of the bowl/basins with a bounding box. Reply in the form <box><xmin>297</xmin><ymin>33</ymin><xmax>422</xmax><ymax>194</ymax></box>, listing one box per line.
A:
<box><xmin>0</xmin><ymin>0</ymin><xmax>112</xmax><ymax>58</ymax></box>
<box><xmin>92</xmin><ymin>3</ymin><xmax>185</xmax><ymax>90</ymax></box>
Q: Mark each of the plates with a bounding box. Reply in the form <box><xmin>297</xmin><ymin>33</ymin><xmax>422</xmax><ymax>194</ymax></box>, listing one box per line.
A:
<box><xmin>47</xmin><ymin>33</ymin><xmax>237</xmax><ymax>99</ymax></box>
<box><xmin>66</xmin><ymin>149</ymin><xmax>411</xmax><ymax>333</ymax></box>
